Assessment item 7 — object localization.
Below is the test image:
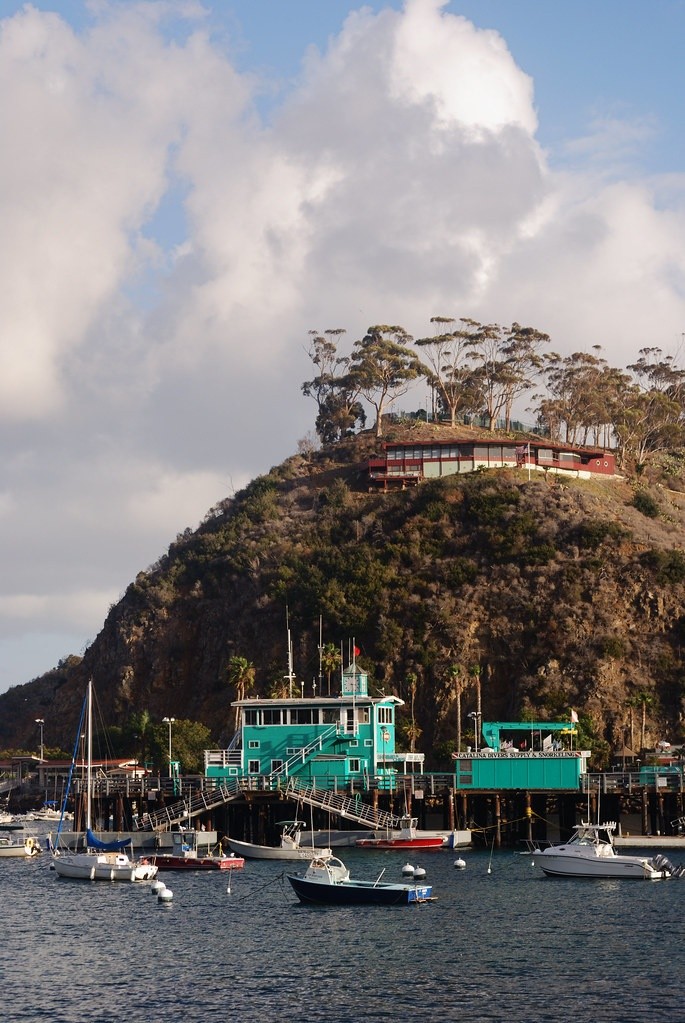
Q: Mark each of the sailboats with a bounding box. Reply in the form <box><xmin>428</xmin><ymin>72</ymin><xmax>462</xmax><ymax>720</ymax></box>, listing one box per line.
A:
<box><xmin>49</xmin><ymin>682</ymin><xmax>158</xmax><ymax>881</ymax></box>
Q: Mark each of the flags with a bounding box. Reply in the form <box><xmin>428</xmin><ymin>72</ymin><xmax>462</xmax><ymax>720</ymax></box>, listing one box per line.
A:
<box><xmin>353</xmin><ymin>645</ymin><xmax>360</xmax><ymax>656</ymax></box>
<box><xmin>570</xmin><ymin>709</ymin><xmax>579</xmax><ymax>723</ymax></box>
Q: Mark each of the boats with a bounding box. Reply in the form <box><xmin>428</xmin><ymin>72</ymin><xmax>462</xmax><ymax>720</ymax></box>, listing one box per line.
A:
<box><xmin>518</xmin><ymin>774</ymin><xmax>685</xmax><ymax>881</ymax></box>
<box><xmin>29</xmin><ymin>800</ymin><xmax>73</xmax><ymax>821</ymax></box>
<box><xmin>137</xmin><ymin>830</ymin><xmax>245</xmax><ymax>871</ymax></box>
<box><xmin>284</xmin><ymin>802</ymin><xmax>438</xmax><ymax>904</ymax></box>
<box><xmin>355</xmin><ymin>780</ymin><xmax>450</xmax><ymax>848</ymax></box>
<box><xmin>225</xmin><ymin>795</ymin><xmax>332</xmax><ymax>861</ymax></box>
<box><xmin>0</xmin><ymin>788</ymin><xmax>35</xmax><ymax>823</ymax></box>
<box><xmin>0</xmin><ymin>821</ymin><xmax>43</xmax><ymax>856</ymax></box>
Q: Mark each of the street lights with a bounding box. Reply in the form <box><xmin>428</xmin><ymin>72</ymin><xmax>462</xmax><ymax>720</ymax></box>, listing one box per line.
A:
<box><xmin>35</xmin><ymin>719</ymin><xmax>44</xmax><ymax>759</ymax></box>
<box><xmin>469</xmin><ymin>712</ymin><xmax>482</xmax><ymax>751</ymax></box>
<box><xmin>162</xmin><ymin>717</ymin><xmax>175</xmax><ymax>779</ymax></box>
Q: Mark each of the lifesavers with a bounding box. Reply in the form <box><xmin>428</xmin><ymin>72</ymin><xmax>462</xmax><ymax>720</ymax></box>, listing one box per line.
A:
<box><xmin>26</xmin><ymin>839</ymin><xmax>33</xmax><ymax>848</ymax></box>
